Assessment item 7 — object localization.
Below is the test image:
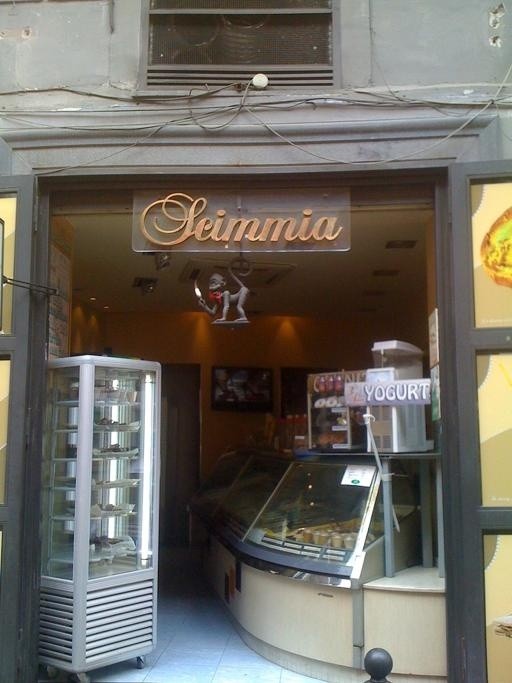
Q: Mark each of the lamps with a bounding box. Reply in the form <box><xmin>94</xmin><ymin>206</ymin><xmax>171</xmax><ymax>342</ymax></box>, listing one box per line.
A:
<box><xmin>234</xmin><ymin>73</ymin><xmax>268</xmax><ymax>89</ymax></box>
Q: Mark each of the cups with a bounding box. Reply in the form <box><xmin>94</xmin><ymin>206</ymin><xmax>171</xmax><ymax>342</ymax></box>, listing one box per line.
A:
<box><xmin>313</xmin><ymin>534</ymin><xmax>319</xmax><ymax>544</ymax></box>
<box><xmin>319</xmin><ymin>534</ymin><xmax>328</xmax><ymax>545</ymax></box>
<box><xmin>304</xmin><ymin>531</ymin><xmax>313</xmax><ymax>542</ymax></box>
<box><xmin>332</xmin><ymin>536</ymin><xmax>343</xmax><ymax>547</ymax></box>
<box><xmin>344</xmin><ymin>538</ymin><xmax>354</xmax><ymax>549</ymax></box>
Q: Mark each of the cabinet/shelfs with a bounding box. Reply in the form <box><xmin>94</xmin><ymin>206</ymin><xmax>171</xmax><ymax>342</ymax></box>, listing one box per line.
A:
<box><xmin>185</xmin><ymin>441</ymin><xmax>446</xmax><ymax>683</ymax></box>
<box><xmin>307</xmin><ymin>370</ymin><xmax>367</xmax><ymax>452</ymax></box>
<box><xmin>38</xmin><ymin>354</ymin><xmax>162</xmax><ymax>682</ymax></box>
